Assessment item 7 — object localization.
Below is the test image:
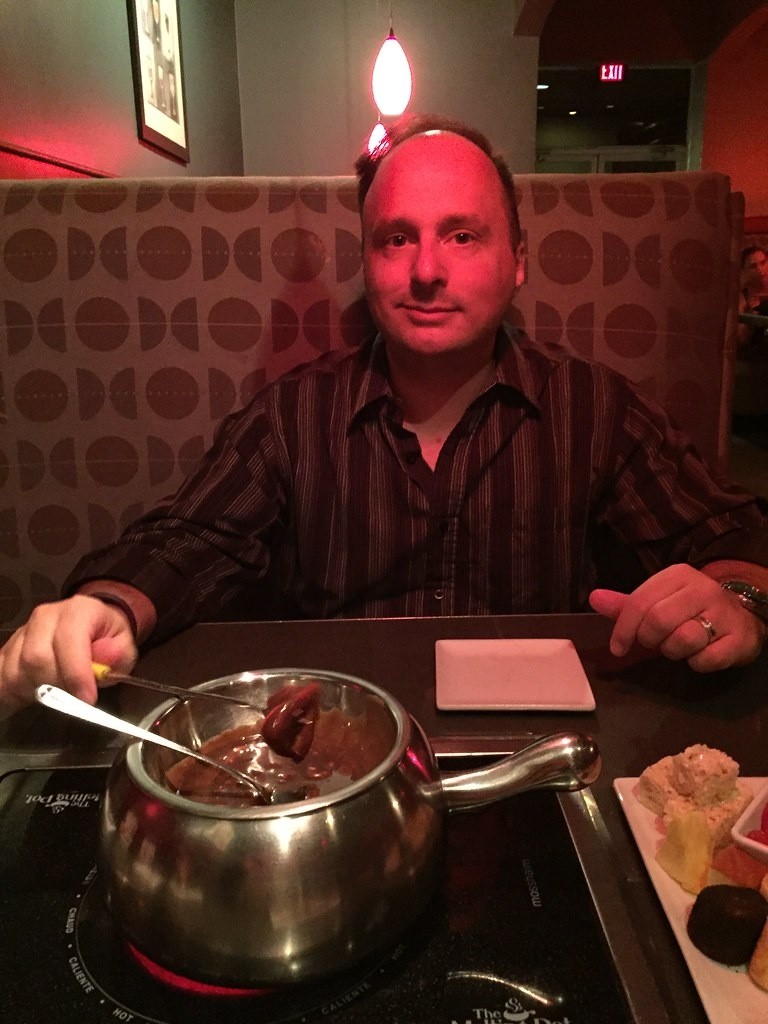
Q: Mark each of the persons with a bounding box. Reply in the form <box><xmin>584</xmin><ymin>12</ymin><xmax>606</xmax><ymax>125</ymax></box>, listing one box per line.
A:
<box><xmin>738</xmin><ymin>246</ymin><xmax>768</xmax><ymax>346</ymax></box>
<box><xmin>0</xmin><ymin>115</ymin><xmax>768</xmax><ymax>720</ymax></box>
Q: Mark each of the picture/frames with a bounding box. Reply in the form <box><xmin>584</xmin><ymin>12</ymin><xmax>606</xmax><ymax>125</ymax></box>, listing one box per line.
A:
<box><xmin>125</xmin><ymin>0</ymin><xmax>193</xmax><ymax>165</ymax></box>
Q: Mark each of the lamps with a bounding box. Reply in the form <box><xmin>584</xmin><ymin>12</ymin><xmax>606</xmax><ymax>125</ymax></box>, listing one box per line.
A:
<box><xmin>366</xmin><ymin>115</ymin><xmax>390</xmax><ymax>158</ymax></box>
<box><xmin>371</xmin><ymin>2</ymin><xmax>414</xmax><ymax>116</ymax></box>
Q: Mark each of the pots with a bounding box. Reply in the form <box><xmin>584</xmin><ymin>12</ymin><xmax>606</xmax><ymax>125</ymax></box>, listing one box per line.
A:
<box><xmin>79</xmin><ymin>669</ymin><xmax>608</xmax><ymax>1005</ymax></box>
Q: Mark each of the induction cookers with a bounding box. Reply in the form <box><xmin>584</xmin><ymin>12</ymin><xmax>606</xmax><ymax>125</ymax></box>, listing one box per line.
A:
<box><xmin>0</xmin><ymin>735</ymin><xmax>668</xmax><ymax>1024</ymax></box>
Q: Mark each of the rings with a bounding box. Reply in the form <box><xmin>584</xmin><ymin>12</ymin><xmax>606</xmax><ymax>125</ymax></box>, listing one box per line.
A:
<box><xmin>692</xmin><ymin>615</ymin><xmax>715</xmax><ymax>645</ymax></box>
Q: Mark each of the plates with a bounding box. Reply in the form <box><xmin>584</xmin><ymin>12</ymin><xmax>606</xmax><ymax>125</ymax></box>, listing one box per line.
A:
<box><xmin>432</xmin><ymin>636</ymin><xmax>596</xmax><ymax>714</ymax></box>
<box><xmin>611</xmin><ymin>773</ymin><xmax>767</xmax><ymax>1024</ymax></box>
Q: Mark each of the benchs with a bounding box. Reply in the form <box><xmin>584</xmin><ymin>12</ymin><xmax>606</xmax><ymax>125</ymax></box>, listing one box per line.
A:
<box><xmin>2</xmin><ymin>169</ymin><xmax>743</xmax><ymax>620</ymax></box>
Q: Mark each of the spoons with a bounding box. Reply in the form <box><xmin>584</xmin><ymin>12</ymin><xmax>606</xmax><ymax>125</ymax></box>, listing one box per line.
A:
<box><xmin>36</xmin><ymin>683</ymin><xmax>305</xmax><ymax>807</ymax></box>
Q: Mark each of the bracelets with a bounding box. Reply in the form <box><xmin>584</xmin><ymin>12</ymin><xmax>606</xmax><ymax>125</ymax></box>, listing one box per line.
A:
<box><xmin>88</xmin><ymin>591</ymin><xmax>137</xmax><ymax>639</ymax></box>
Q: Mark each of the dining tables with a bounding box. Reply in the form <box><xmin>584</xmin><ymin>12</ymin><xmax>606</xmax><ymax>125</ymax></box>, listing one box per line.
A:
<box><xmin>1</xmin><ymin>608</ymin><xmax>767</xmax><ymax>1024</ymax></box>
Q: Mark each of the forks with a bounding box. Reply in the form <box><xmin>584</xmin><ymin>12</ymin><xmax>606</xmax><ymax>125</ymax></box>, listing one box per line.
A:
<box><xmin>89</xmin><ymin>661</ymin><xmax>317</xmax><ymax>730</ymax></box>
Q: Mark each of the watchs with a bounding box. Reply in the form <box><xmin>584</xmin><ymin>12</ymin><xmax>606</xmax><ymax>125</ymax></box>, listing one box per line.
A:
<box><xmin>720</xmin><ymin>580</ymin><xmax>767</xmax><ymax>620</ymax></box>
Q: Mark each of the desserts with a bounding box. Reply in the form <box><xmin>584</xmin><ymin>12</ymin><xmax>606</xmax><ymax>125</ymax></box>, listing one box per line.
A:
<box><xmin>632</xmin><ymin>744</ymin><xmax>767</xmax><ymax>993</ymax></box>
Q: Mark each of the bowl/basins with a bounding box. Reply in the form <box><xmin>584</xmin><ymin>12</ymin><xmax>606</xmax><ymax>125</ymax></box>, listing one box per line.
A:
<box><xmin>730</xmin><ymin>784</ymin><xmax>767</xmax><ymax>863</ymax></box>
